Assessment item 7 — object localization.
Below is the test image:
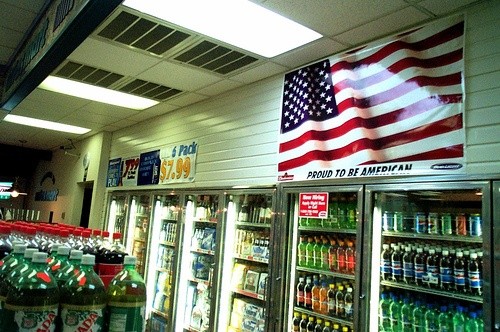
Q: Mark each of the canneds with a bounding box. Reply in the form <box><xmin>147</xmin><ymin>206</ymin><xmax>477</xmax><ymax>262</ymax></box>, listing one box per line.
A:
<box><xmin>383</xmin><ymin>210</ymin><xmax>483</xmax><ymax>238</ymax></box>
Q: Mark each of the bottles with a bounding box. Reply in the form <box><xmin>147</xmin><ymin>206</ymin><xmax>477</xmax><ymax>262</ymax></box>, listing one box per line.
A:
<box><xmin>0</xmin><ymin>208</ymin><xmax>148</xmax><ymax>332</ymax></box>
<box><xmin>109</xmin><ymin>190</ymin><xmax>488</xmax><ymax>332</ymax></box>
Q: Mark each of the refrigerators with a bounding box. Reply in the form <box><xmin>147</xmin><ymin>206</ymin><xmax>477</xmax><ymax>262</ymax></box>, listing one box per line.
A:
<box><xmin>100</xmin><ymin>171</ymin><xmax>499</xmax><ymax>331</ymax></box>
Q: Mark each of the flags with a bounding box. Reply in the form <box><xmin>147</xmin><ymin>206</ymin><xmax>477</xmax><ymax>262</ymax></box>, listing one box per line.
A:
<box><xmin>276</xmin><ymin>11</ymin><xmax>468</xmax><ymax>182</ymax></box>
